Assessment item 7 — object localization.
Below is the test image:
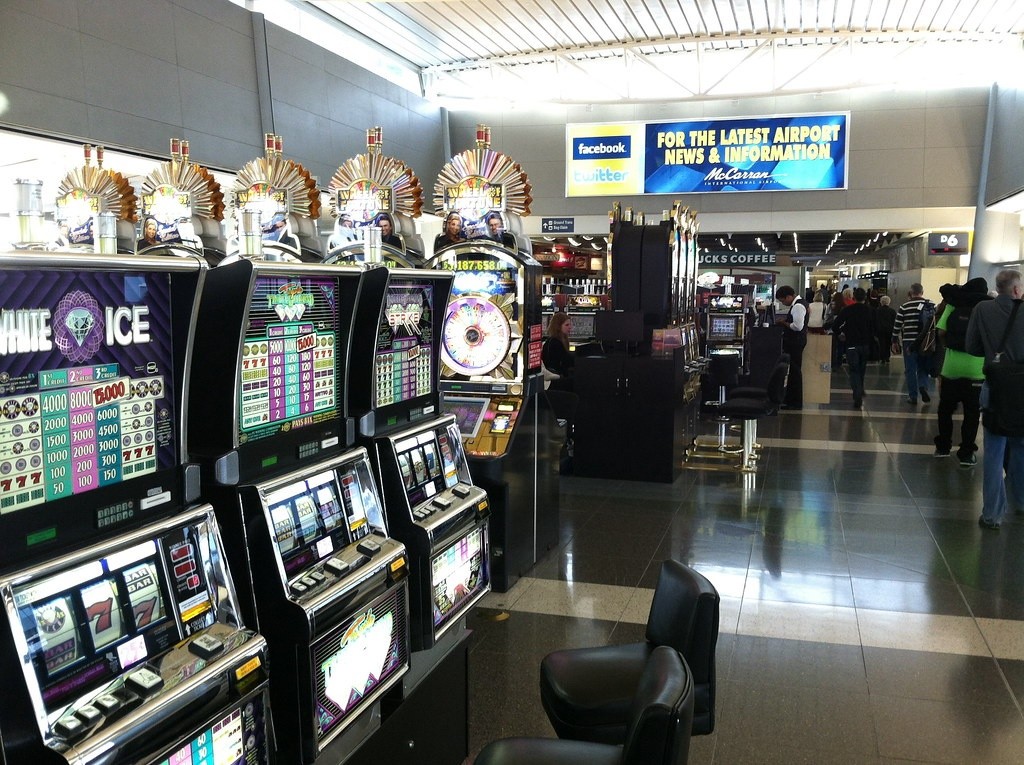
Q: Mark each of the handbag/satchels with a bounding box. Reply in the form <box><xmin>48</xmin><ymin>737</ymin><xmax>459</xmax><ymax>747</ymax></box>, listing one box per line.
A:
<box><xmin>918</xmin><ymin>314</ymin><xmax>936</xmax><ymax>356</ymax></box>
<box><xmin>982</xmin><ymin>351</ymin><xmax>1014</xmax><ymax>383</ymax></box>
<box><xmin>823</xmin><ymin>303</ymin><xmax>835</xmax><ymax>330</ymax></box>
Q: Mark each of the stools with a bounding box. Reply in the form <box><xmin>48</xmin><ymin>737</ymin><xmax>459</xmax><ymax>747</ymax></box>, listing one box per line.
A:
<box><xmin>474</xmin><ymin>647</ymin><xmax>695</xmax><ymax>764</ymax></box>
<box><xmin>685</xmin><ymin>353</ymin><xmax>791</xmax><ymax>472</ymax></box>
<box><xmin>541</xmin><ymin>559</ymin><xmax>720</xmax><ymax>746</ymax></box>
<box><xmin>545</xmin><ymin>389</ymin><xmax>576</xmax><ymax>464</ymax></box>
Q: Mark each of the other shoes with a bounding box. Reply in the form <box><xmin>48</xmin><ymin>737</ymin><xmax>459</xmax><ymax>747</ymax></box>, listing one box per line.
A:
<box><xmin>881</xmin><ymin>360</ymin><xmax>885</xmax><ymax>364</ymax></box>
<box><xmin>919</xmin><ymin>387</ymin><xmax>931</xmax><ymax>403</ymax></box>
<box><xmin>886</xmin><ymin>358</ymin><xmax>890</xmax><ymax>362</ymax></box>
<box><xmin>959</xmin><ymin>453</ymin><xmax>978</xmax><ymax>466</ymax></box>
<box><xmin>855</xmin><ymin>400</ymin><xmax>862</xmax><ymax>408</ymax></box>
<box><xmin>979</xmin><ymin>515</ymin><xmax>1000</xmax><ymax>530</ymax></box>
<box><xmin>780</xmin><ymin>405</ymin><xmax>802</xmax><ymax>410</ymax></box>
<box><xmin>933</xmin><ymin>450</ymin><xmax>950</xmax><ymax>457</ymax></box>
<box><xmin>906</xmin><ymin>398</ymin><xmax>917</xmax><ymax>405</ymax></box>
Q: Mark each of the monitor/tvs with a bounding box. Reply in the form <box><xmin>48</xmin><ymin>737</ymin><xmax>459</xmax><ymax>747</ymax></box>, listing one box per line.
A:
<box><xmin>238</xmin><ymin>275</ymin><xmax>342</xmax><ymax>445</ymax></box>
<box><xmin>595</xmin><ymin>310</ymin><xmax>644</xmax><ymax>343</ymax></box>
<box><xmin>376</xmin><ymin>280</ymin><xmax>434</xmax><ymax>408</ymax></box>
<box><xmin>0</xmin><ymin>268</ymin><xmax>178</xmax><ymax>515</ymax></box>
<box><xmin>708</xmin><ymin>314</ymin><xmax>742</xmax><ymax>338</ymax></box>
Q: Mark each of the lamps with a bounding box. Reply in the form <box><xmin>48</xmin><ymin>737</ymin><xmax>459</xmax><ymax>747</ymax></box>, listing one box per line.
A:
<box><xmin>703</xmin><ymin>231</ymin><xmax>888</xmax><ymax>268</ymax></box>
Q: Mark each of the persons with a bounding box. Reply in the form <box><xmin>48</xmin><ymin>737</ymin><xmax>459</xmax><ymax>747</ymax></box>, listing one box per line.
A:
<box><xmin>487</xmin><ymin>213</ymin><xmax>516</xmax><ymax>249</ymax></box>
<box><xmin>806</xmin><ymin>284</ymin><xmax>897</xmax><ymax>408</ymax></box>
<box><xmin>376</xmin><ymin>214</ymin><xmax>402</xmax><ymax>249</ymax></box>
<box><xmin>434</xmin><ymin>213</ymin><xmax>467</xmax><ymax>253</ymax></box>
<box><xmin>541</xmin><ymin>312</ymin><xmax>576</xmax><ymax>404</ymax></box>
<box><xmin>966</xmin><ymin>270</ymin><xmax>1024</xmax><ymax>529</ymax></box>
<box><xmin>137</xmin><ymin>218</ymin><xmax>158</xmax><ymax>251</ymax></box>
<box><xmin>934</xmin><ymin>278</ymin><xmax>994</xmax><ymax>465</ymax></box>
<box><xmin>776</xmin><ymin>286</ymin><xmax>809</xmax><ymax>410</ymax></box>
<box><xmin>892</xmin><ymin>283</ymin><xmax>935</xmax><ymax>403</ymax></box>
